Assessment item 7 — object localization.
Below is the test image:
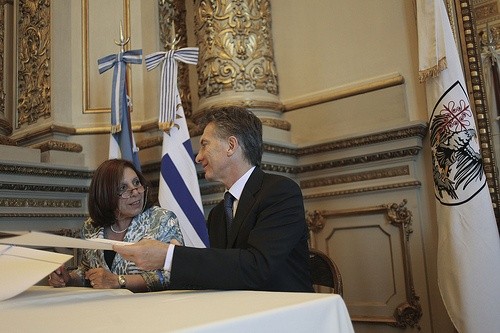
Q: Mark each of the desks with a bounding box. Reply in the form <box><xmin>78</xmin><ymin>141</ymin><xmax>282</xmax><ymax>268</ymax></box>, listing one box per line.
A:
<box><xmin>0</xmin><ymin>286</ymin><xmax>355</xmax><ymax>333</ymax></box>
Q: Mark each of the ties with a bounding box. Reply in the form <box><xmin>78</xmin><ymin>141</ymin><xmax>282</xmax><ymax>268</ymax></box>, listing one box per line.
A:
<box><xmin>223</xmin><ymin>191</ymin><xmax>236</xmax><ymax>245</ymax></box>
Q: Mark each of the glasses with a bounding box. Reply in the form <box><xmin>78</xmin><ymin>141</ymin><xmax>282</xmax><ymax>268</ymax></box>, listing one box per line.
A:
<box><xmin>116</xmin><ymin>181</ymin><xmax>145</xmax><ymax>199</ymax></box>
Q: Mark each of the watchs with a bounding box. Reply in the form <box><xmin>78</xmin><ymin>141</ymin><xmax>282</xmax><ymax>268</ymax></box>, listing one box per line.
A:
<box><xmin>118</xmin><ymin>275</ymin><xmax>126</xmax><ymax>289</ymax></box>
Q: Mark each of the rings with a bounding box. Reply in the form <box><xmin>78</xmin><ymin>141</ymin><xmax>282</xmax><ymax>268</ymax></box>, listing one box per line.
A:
<box><xmin>48</xmin><ymin>276</ymin><xmax>51</xmax><ymax>280</ymax></box>
<box><xmin>90</xmin><ymin>281</ymin><xmax>94</xmax><ymax>287</ymax></box>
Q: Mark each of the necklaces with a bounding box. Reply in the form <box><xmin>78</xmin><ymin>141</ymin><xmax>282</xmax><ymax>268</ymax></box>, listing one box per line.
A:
<box><xmin>110</xmin><ymin>226</ymin><xmax>127</xmax><ymax>234</ymax></box>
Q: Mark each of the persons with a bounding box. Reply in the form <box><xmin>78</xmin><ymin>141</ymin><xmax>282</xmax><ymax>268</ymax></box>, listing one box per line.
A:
<box><xmin>113</xmin><ymin>106</ymin><xmax>317</xmax><ymax>293</ymax></box>
<box><xmin>47</xmin><ymin>158</ymin><xmax>185</xmax><ymax>292</ymax></box>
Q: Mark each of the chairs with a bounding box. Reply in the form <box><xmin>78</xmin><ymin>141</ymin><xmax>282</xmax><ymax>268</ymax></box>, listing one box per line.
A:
<box><xmin>308</xmin><ymin>248</ymin><xmax>343</xmax><ymax>298</ymax></box>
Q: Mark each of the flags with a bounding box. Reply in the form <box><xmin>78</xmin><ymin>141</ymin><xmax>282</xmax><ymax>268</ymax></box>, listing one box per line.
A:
<box><xmin>96</xmin><ymin>48</ymin><xmax>144</xmax><ymax>173</ymax></box>
<box><xmin>417</xmin><ymin>0</ymin><xmax>500</xmax><ymax>333</ymax></box>
<box><xmin>144</xmin><ymin>47</ymin><xmax>210</xmax><ymax>250</ymax></box>
